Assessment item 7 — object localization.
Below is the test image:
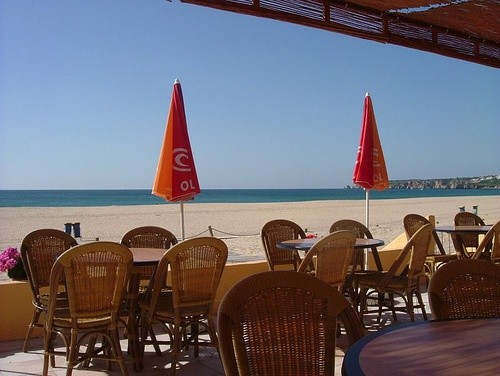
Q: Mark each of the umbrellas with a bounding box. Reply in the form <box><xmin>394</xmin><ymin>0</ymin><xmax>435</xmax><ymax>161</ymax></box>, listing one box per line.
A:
<box><xmin>352</xmin><ymin>91</ymin><xmax>390</xmax><ymax>270</ymax></box>
<box><xmin>151</xmin><ymin>78</ymin><xmax>202</xmax><ymax>242</ymax></box>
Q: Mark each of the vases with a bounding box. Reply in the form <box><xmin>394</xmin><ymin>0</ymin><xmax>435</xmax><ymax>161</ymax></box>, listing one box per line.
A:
<box><xmin>7</xmin><ymin>259</ymin><xmax>27</xmax><ymax>280</ymax></box>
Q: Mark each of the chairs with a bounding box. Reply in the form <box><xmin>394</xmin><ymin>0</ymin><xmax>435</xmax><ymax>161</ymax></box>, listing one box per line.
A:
<box><xmin>470</xmin><ymin>220</ymin><xmax>499</xmax><ymax>266</ymax></box>
<box><xmin>43</xmin><ymin>241</ymin><xmax>134</xmax><ymax>375</ymax></box>
<box><xmin>217</xmin><ymin>270</ymin><xmax>365</xmax><ymax>376</ymax></box>
<box><xmin>329</xmin><ymin>220</ymin><xmax>383</xmax><ymax>338</ymax></box>
<box><xmin>403</xmin><ymin>214</ymin><xmax>456</xmax><ymax>280</ymax></box>
<box><xmin>359</xmin><ymin>213</ymin><xmax>428</xmax><ymax>329</ymax></box>
<box><xmin>138</xmin><ymin>237</ymin><xmax>219</xmax><ymax>376</ymax></box>
<box><xmin>296</xmin><ymin>230</ymin><xmax>356</xmax><ymax>337</ymax></box>
<box><xmin>427</xmin><ymin>259</ymin><xmax>499</xmax><ymax>320</ymax></box>
<box><xmin>453</xmin><ymin>212</ymin><xmax>490</xmax><ymax>261</ymax></box>
<box><xmin>262</xmin><ymin>219</ymin><xmax>315</xmax><ymax>276</ymax></box>
<box><xmin>121</xmin><ymin>226</ymin><xmax>189</xmax><ymax>356</ymax></box>
<box><xmin>20</xmin><ymin>228</ymin><xmax>105</xmax><ymax>368</ymax></box>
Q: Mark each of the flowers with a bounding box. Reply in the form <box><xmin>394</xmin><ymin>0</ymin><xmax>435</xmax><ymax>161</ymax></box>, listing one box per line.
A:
<box><xmin>0</xmin><ymin>246</ymin><xmax>20</xmax><ymax>272</ymax></box>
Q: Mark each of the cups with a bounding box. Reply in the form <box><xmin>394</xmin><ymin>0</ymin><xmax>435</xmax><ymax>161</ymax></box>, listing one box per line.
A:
<box><xmin>73</xmin><ymin>222</ymin><xmax>80</xmax><ymax>237</ymax></box>
<box><xmin>472</xmin><ymin>205</ymin><xmax>478</xmax><ymax>214</ymax></box>
<box><xmin>65</xmin><ymin>222</ymin><xmax>72</xmax><ymax>236</ymax></box>
<box><xmin>459</xmin><ymin>206</ymin><xmax>466</xmax><ymax>214</ymax></box>
